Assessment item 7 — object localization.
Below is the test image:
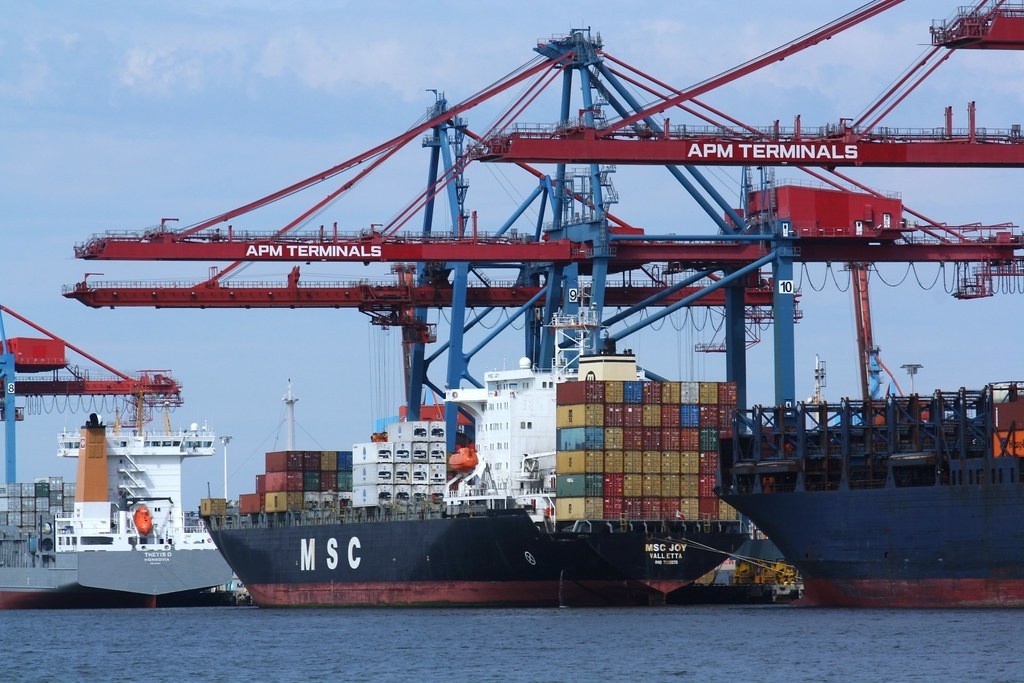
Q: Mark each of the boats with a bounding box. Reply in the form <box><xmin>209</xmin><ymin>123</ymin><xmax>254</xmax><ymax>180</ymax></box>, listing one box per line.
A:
<box><xmin>133</xmin><ymin>506</ymin><xmax>153</xmax><ymax>537</ymax></box>
<box><xmin>199</xmin><ymin>305</ymin><xmax>1023</xmax><ymax>617</ymax></box>
<box><xmin>446</xmin><ymin>446</ymin><xmax>480</xmax><ymax>476</ymax></box>
<box><xmin>0</xmin><ymin>392</ymin><xmax>235</xmax><ymax>611</ymax></box>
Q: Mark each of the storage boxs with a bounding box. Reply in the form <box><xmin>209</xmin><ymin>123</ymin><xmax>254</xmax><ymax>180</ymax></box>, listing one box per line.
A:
<box><xmin>1</xmin><ymin>476</ymin><xmax>76</xmax><ymax>531</ymax></box>
<box><xmin>554</xmin><ymin>381</ymin><xmax>736</xmax><ymax>519</ymax></box>
<box><xmin>201</xmin><ymin>419</ymin><xmax>448</xmax><ymax>515</ymax></box>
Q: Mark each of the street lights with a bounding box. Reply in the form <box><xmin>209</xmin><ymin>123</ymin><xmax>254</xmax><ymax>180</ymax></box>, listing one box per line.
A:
<box><xmin>218</xmin><ymin>435</ymin><xmax>233</xmax><ymax>506</ymax></box>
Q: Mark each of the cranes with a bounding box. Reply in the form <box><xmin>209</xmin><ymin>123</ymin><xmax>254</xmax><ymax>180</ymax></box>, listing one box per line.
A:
<box><xmin>1</xmin><ymin>307</ymin><xmax>183</xmax><ymax>437</ymax></box>
<box><xmin>61</xmin><ymin>0</ymin><xmax>1024</xmax><ymax>450</ymax></box>
